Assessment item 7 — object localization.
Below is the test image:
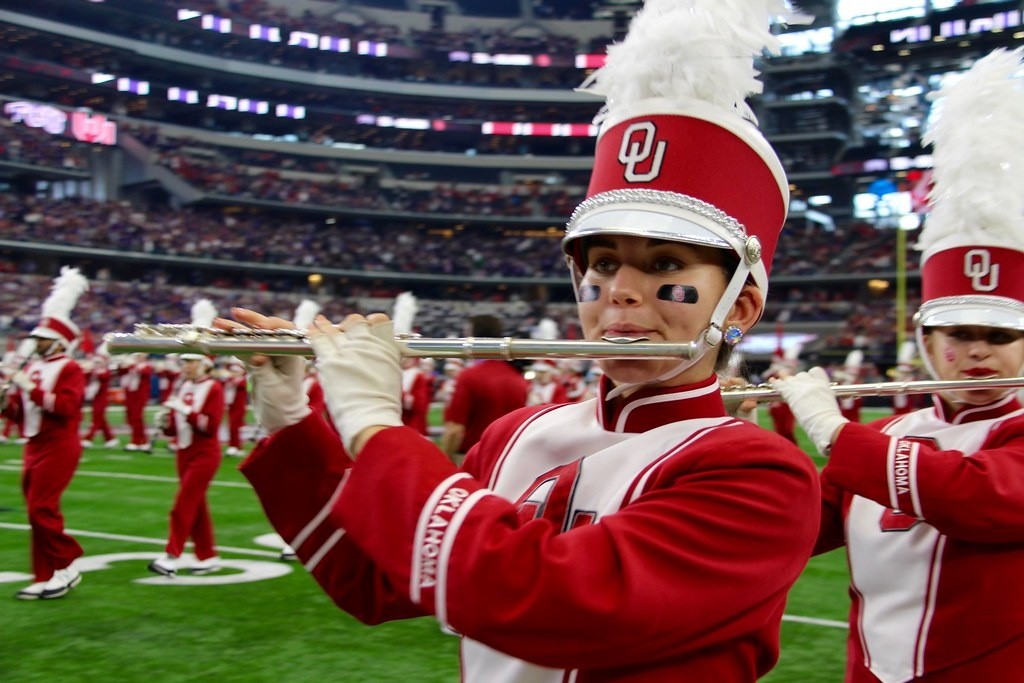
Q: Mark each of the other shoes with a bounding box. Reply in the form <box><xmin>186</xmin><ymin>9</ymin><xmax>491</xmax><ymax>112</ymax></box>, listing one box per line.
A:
<box><xmin>227</xmin><ymin>447</ymin><xmax>242</xmax><ymax>456</ymax></box>
<box><xmin>105</xmin><ymin>438</ymin><xmax>119</xmax><ymax>448</ymax></box>
<box><xmin>16</xmin><ymin>438</ymin><xmax>27</xmax><ymax>443</ymax></box>
<box><xmin>81</xmin><ymin>438</ymin><xmax>91</xmax><ymax>444</ymax></box>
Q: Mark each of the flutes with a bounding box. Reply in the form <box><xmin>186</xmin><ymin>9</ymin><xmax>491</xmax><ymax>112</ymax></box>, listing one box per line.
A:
<box><xmin>0</xmin><ymin>344</ymin><xmax>39</xmax><ymax>404</ymax></box>
<box><xmin>717</xmin><ymin>365</ymin><xmax>1024</xmax><ymax>408</ymax></box>
<box><xmin>143</xmin><ymin>371</ymin><xmax>186</xmax><ymax>453</ymax></box>
<box><xmin>88</xmin><ymin>314</ymin><xmax>704</xmax><ymax>369</ymax></box>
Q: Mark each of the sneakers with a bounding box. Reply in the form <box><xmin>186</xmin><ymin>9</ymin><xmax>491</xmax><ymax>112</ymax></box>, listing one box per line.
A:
<box><xmin>125</xmin><ymin>441</ymin><xmax>152</xmax><ymax>453</ymax></box>
<box><xmin>15</xmin><ymin>567</ymin><xmax>83</xmax><ymax>600</ymax></box>
<box><xmin>148</xmin><ymin>556</ymin><xmax>177</xmax><ymax>578</ymax></box>
<box><xmin>282</xmin><ymin>545</ymin><xmax>297</xmax><ymax>558</ymax></box>
<box><xmin>191</xmin><ymin>558</ymin><xmax>221</xmax><ymax>574</ymax></box>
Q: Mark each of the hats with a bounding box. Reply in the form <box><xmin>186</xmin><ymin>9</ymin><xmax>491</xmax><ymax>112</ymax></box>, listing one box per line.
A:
<box><xmin>560</xmin><ymin>0</ymin><xmax>817</xmax><ymax>326</ymax></box>
<box><xmin>444</xmin><ymin>359</ymin><xmax>465</xmax><ymax>371</ymax></box>
<box><xmin>531</xmin><ymin>358</ymin><xmax>556</xmax><ymax>373</ymax></box>
<box><xmin>914</xmin><ymin>45</ymin><xmax>1023</xmax><ymax>333</ymax></box>
<box><xmin>17</xmin><ymin>266</ymin><xmax>89</xmax><ymax>349</ymax></box>
<box><xmin>180</xmin><ymin>302</ymin><xmax>217</xmax><ymax>368</ymax></box>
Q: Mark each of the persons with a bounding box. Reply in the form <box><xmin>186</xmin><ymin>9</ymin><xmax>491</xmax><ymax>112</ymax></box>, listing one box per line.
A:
<box><xmin>1</xmin><ymin>0</ymin><xmax>987</xmax><ymax>356</ymax></box>
<box><xmin>443</xmin><ymin>345</ymin><xmax>590</xmax><ymax>417</ymax></box>
<box><xmin>149</xmin><ymin>343</ymin><xmax>224</xmax><ymax>578</ymax></box>
<box><xmin>756</xmin><ymin>360</ymin><xmax>924</xmax><ymax>452</ymax></box>
<box><xmin>1</xmin><ymin>312</ymin><xmax>88</xmax><ymax>599</ymax></box>
<box><xmin>212</xmin><ymin>99</ymin><xmax>822</xmax><ymax>683</ymax></box>
<box><xmin>1</xmin><ymin>336</ymin><xmax>439</xmax><ymax>460</ymax></box>
<box><xmin>436</xmin><ymin>315</ymin><xmax>528</xmax><ymax>471</ymax></box>
<box><xmin>716</xmin><ymin>224</ymin><xmax>1024</xmax><ymax>683</ymax></box>
